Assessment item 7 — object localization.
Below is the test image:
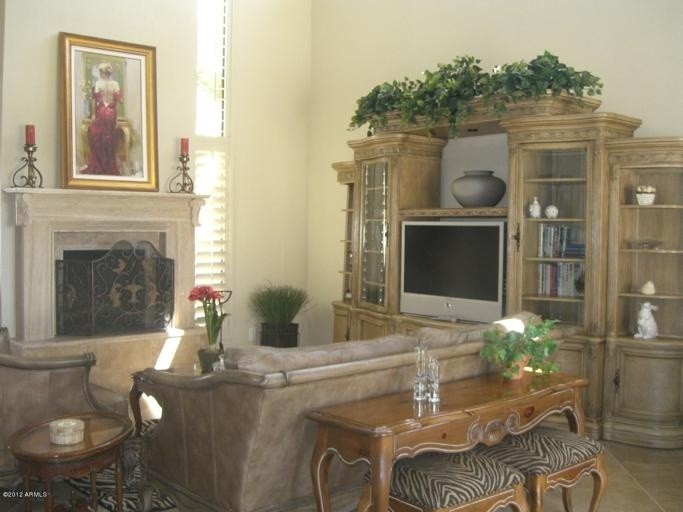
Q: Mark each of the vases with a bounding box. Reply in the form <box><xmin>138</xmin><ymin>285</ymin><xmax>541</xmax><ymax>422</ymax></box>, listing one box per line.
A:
<box><xmin>449</xmin><ymin>169</ymin><xmax>506</xmax><ymax>209</ymax></box>
<box><xmin>198</xmin><ymin>348</ymin><xmax>229</xmax><ymax>374</ymax></box>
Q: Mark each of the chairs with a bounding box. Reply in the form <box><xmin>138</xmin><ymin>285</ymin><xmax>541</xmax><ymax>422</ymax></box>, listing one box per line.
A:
<box><xmin>0</xmin><ymin>327</ymin><xmax>130</xmax><ymax>505</ymax></box>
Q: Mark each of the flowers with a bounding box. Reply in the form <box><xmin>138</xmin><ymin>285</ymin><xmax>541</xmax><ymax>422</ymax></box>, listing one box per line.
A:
<box><xmin>188</xmin><ymin>286</ymin><xmax>231</xmax><ymax>350</ymax></box>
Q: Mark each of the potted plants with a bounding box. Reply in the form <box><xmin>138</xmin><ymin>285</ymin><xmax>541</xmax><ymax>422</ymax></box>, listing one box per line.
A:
<box><xmin>248</xmin><ymin>286</ymin><xmax>310</xmax><ymax>349</ymax></box>
<box><xmin>478</xmin><ymin>315</ymin><xmax>562</xmax><ymax>380</ymax></box>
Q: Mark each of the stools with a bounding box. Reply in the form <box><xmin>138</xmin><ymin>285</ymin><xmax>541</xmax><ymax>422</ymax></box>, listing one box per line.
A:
<box><xmin>473</xmin><ymin>426</ymin><xmax>608</xmax><ymax>512</ymax></box>
<box><xmin>356</xmin><ymin>451</ymin><xmax>533</xmax><ymax>512</ymax></box>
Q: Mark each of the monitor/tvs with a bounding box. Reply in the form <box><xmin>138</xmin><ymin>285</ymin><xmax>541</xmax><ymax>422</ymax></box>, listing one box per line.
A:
<box><xmin>399</xmin><ymin>220</ymin><xmax>506</xmax><ymax>324</ymax></box>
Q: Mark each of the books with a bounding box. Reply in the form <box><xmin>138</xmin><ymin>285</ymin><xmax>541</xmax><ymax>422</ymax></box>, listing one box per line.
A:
<box><xmin>535</xmin><ymin>222</ymin><xmax>583</xmax><ymax>300</ymax></box>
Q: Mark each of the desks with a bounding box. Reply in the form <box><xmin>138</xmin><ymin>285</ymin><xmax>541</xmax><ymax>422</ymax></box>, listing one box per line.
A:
<box><xmin>303</xmin><ymin>371</ymin><xmax>592</xmax><ymax>512</ymax></box>
<box><xmin>7</xmin><ymin>411</ymin><xmax>135</xmax><ymax>512</ymax></box>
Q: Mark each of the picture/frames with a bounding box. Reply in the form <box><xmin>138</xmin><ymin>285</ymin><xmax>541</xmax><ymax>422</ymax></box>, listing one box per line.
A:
<box><xmin>58</xmin><ymin>32</ymin><xmax>160</xmax><ymax>192</ymax></box>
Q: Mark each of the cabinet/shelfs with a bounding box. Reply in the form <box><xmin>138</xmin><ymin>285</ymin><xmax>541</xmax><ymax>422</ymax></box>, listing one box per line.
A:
<box><xmin>331</xmin><ymin>161</ymin><xmax>361</xmax><ymax>343</ymax></box>
<box><xmin>602</xmin><ymin>139</ymin><xmax>683</xmax><ymax>449</ymax></box>
<box><xmin>346</xmin><ymin>134</ymin><xmax>447</xmax><ymax>340</ymax></box>
<box><xmin>499</xmin><ymin>112</ymin><xmax>643</xmax><ymax>439</ymax></box>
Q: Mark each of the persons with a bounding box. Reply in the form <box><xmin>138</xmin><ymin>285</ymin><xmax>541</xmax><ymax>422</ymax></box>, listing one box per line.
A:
<box><xmin>76</xmin><ymin>59</ymin><xmax>128</xmax><ymax>179</ymax></box>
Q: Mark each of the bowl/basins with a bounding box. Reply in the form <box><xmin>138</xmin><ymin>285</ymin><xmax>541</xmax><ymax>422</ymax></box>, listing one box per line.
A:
<box><xmin>48</xmin><ymin>418</ymin><xmax>85</xmax><ymax>445</ymax></box>
<box><xmin>50</xmin><ymin>445</ymin><xmax>84</xmax><ymax>453</ymax></box>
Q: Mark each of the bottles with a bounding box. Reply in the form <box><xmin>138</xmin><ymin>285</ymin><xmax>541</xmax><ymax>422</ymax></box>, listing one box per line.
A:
<box><xmin>530</xmin><ymin>196</ymin><xmax>541</xmax><ymax>220</ymax></box>
<box><xmin>635</xmin><ymin>183</ymin><xmax>657</xmax><ymax>206</ymax></box>
<box><xmin>411</xmin><ymin>403</ymin><xmax>439</xmax><ymax>417</ymax></box>
<box><xmin>412</xmin><ymin>347</ymin><xmax>441</xmax><ymax>403</ymax></box>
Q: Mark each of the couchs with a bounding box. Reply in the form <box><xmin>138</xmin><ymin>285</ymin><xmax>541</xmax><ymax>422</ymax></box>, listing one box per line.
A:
<box><xmin>129</xmin><ymin>311</ymin><xmax>568</xmax><ymax>512</ymax></box>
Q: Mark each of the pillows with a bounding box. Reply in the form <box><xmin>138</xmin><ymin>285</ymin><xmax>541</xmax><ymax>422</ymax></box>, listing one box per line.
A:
<box><xmin>413</xmin><ymin>311</ymin><xmax>544</xmax><ymax>349</ymax></box>
<box><xmin>223</xmin><ymin>333</ymin><xmax>420</xmax><ymax>374</ymax></box>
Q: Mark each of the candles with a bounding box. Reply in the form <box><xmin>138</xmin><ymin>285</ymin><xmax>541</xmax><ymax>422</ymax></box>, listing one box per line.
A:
<box><xmin>181</xmin><ymin>138</ymin><xmax>189</xmax><ymax>155</ymax></box>
<box><xmin>26</xmin><ymin>125</ymin><xmax>35</xmax><ymax>145</ymax></box>
